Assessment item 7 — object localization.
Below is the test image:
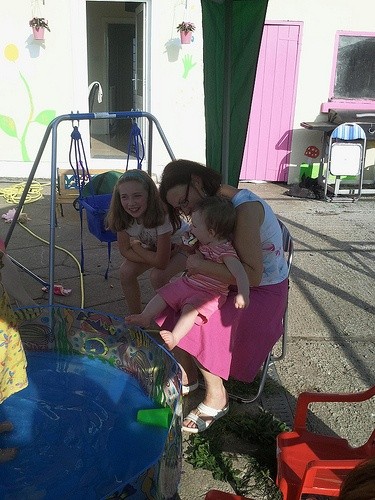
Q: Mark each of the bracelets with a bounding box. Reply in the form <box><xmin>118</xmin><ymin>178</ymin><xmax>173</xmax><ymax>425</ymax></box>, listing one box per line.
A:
<box><xmin>130</xmin><ymin>240</ymin><xmax>142</xmax><ymax>248</ymax></box>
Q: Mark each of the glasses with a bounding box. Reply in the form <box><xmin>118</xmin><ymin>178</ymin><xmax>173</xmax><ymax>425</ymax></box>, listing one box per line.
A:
<box><xmin>175</xmin><ymin>183</ymin><xmax>190</xmax><ymax>212</ymax></box>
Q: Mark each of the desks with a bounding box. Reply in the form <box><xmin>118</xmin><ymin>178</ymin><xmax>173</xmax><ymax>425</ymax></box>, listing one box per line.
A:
<box><xmin>301</xmin><ymin>121</ymin><xmax>375</xmax><ymax>201</ymax></box>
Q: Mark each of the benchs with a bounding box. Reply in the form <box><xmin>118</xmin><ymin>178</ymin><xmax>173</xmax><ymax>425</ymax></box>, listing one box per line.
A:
<box><xmin>55</xmin><ymin>168</ymin><xmax>129</xmax><ymax>227</ymax></box>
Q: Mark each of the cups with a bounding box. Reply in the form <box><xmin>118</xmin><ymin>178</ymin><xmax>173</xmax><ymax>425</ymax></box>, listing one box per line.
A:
<box><xmin>136</xmin><ymin>408</ymin><xmax>171</xmax><ymax>428</ymax></box>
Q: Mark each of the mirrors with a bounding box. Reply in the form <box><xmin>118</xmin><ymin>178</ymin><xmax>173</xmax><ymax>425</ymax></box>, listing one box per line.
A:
<box><xmin>328</xmin><ymin>30</ymin><xmax>375</xmax><ymax>104</ymax></box>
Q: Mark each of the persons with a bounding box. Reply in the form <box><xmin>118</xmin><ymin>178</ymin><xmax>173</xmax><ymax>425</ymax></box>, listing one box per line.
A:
<box><xmin>159</xmin><ymin>159</ymin><xmax>288</xmax><ymax>434</ymax></box>
<box><xmin>104</xmin><ymin>169</ymin><xmax>192</xmax><ymax>329</ymax></box>
<box><xmin>125</xmin><ymin>196</ymin><xmax>251</xmax><ymax>350</ymax></box>
<box><xmin>0</xmin><ymin>251</ymin><xmax>30</xmax><ymax>464</ymax></box>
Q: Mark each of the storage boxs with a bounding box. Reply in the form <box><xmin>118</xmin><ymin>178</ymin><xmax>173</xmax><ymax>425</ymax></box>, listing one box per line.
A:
<box><xmin>77</xmin><ymin>194</ymin><xmax>119</xmax><ymax>242</ymax></box>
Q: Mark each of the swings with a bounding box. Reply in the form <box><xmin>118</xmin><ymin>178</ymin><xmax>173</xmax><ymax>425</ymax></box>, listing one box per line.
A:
<box><xmin>67</xmin><ymin>118</ymin><xmax>144</xmax><ymax>246</ymax></box>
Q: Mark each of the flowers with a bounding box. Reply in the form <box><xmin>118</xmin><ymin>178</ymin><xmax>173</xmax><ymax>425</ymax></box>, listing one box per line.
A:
<box><xmin>176</xmin><ymin>21</ymin><xmax>196</xmax><ymax>37</ymax></box>
<box><xmin>29</xmin><ymin>17</ymin><xmax>51</xmax><ymax>32</ymax></box>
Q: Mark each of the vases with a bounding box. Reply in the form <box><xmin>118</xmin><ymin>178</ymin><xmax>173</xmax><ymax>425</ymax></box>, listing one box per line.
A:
<box><xmin>180</xmin><ymin>31</ymin><xmax>192</xmax><ymax>44</ymax></box>
<box><xmin>32</xmin><ymin>26</ymin><xmax>45</xmax><ymax>40</ymax></box>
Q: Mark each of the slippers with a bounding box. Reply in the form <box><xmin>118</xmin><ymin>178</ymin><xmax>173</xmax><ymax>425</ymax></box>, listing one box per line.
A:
<box><xmin>180</xmin><ymin>400</ymin><xmax>229</xmax><ymax>434</ymax></box>
<box><xmin>181</xmin><ymin>378</ymin><xmax>201</xmax><ymax>394</ymax></box>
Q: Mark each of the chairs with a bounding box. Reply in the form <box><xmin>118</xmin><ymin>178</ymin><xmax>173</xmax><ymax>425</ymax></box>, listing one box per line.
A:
<box><xmin>275</xmin><ymin>385</ymin><xmax>375</xmax><ymax>500</ymax></box>
<box><xmin>198</xmin><ymin>217</ymin><xmax>294</xmax><ymax>402</ymax></box>
<box><xmin>324</xmin><ymin>123</ymin><xmax>366</xmax><ymax>202</ymax></box>
